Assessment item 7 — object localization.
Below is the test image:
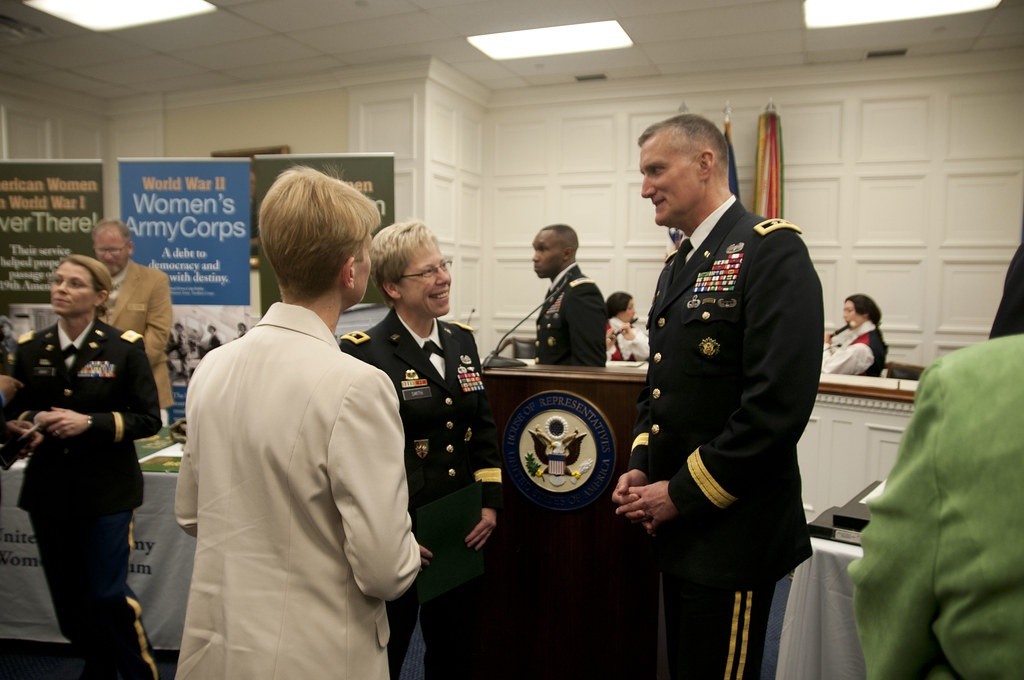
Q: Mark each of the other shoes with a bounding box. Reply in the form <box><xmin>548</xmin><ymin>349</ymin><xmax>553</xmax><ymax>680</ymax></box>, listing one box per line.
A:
<box><xmin>79</xmin><ymin>659</ymin><xmax>118</xmax><ymax>680</ymax></box>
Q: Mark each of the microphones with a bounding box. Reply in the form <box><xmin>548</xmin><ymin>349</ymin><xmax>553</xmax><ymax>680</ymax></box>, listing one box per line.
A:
<box><xmin>482</xmin><ymin>272</ymin><xmax>572</xmax><ymax>368</ymax></box>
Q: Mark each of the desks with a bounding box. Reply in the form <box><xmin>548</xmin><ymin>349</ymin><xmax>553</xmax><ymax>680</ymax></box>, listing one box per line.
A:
<box><xmin>633</xmin><ymin>362</ymin><xmax>920</xmax><ymax>524</ymax></box>
<box><xmin>0</xmin><ymin>426</ymin><xmax>196</xmax><ymax>650</ymax></box>
<box><xmin>776</xmin><ymin>480</ymin><xmax>881</xmax><ymax>679</ymax></box>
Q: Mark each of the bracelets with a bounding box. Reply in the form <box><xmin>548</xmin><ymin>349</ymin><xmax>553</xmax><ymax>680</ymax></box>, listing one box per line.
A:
<box><xmin>88</xmin><ymin>415</ymin><xmax>94</xmax><ymax>424</ymax></box>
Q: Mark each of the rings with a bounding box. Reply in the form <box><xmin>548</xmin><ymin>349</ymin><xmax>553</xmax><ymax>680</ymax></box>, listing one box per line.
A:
<box><xmin>55</xmin><ymin>430</ymin><xmax>60</xmax><ymax>436</ymax></box>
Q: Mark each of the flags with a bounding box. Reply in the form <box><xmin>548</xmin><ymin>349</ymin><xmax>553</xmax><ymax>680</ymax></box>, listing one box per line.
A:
<box><xmin>754</xmin><ymin>113</ymin><xmax>785</xmax><ymax>219</ymax></box>
<box><xmin>722</xmin><ymin>124</ymin><xmax>739</xmax><ymax>199</ymax></box>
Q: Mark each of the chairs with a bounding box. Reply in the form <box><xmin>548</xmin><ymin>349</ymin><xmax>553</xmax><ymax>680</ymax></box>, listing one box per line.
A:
<box><xmin>882</xmin><ymin>363</ymin><xmax>925</xmax><ymax>380</ymax></box>
<box><xmin>512</xmin><ymin>337</ymin><xmax>538</xmax><ymax>359</ymax></box>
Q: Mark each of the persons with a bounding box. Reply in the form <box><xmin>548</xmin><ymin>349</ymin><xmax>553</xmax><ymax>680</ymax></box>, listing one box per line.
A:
<box><xmin>0</xmin><ymin>254</ymin><xmax>159</xmax><ymax>680</ymax></box>
<box><xmin>606</xmin><ymin>292</ymin><xmax>650</xmax><ymax>362</ymax></box>
<box><xmin>92</xmin><ymin>216</ymin><xmax>175</xmax><ymax>409</ymax></box>
<box><xmin>532</xmin><ymin>224</ymin><xmax>607</xmax><ymax>367</ymax></box>
<box><xmin>174</xmin><ymin>164</ymin><xmax>420</xmax><ymax>680</ymax></box>
<box><xmin>339</xmin><ymin>223</ymin><xmax>502</xmax><ymax>680</ymax></box>
<box><xmin>822</xmin><ymin>294</ymin><xmax>888</xmax><ymax>376</ymax></box>
<box><xmin>238</xmin><ymin>323</ymin><xmax>246</xmax><ymax>337</ymax></box>
<box><xmin>207</xmin><ymin>325</ymin><xmax>220</xmax><ymax>349</ymax></box>
<box><xmin>611</xmin><ymin>116</ymin><xmax>825</xmax><ymax>680</ymax></box>
<box><xmin>849</xmin><ymin>229</ymin><xmax>1024</xmax><ymax>680</ymax></box>
<box><xmin>164</xmin><ymin>322</ymin><xmax>189</xmax><ymax>379</ymax></box>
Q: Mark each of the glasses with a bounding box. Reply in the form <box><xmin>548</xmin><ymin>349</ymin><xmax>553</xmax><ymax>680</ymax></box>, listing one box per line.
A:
<box><xmin>400</xmin><ymin>259</ymin><xmax>452</xmax><ymax>278</ymax></box>
<box><xmin>94</xmin><ymin>241</ymin><xmax>128</xmax><ymax>255</ymax></box>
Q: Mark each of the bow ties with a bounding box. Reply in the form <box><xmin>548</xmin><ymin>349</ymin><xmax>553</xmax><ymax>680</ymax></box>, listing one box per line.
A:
<box><xmin>423</xmin><ymin>340</ymin><xmax>445</xmax><ymax>360</ymax></box>
<box><xmin>63</xmin><ymin>346</ymin><xmax>80</xmax><ymax>359</ymax></box>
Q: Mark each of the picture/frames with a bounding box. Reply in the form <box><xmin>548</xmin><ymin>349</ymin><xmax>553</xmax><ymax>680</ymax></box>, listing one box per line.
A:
<box><xmin>209</xmin><ymin>143</ymin><xmax>291</xmax><ymax>268</ymax></box>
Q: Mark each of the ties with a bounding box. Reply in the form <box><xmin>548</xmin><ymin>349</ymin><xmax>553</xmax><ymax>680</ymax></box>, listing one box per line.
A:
<box><xmin>664</xmin><ymin>238</ymin><xmax>693</xmax><ymax>299</ymax></box>
<box><xmin>545</xmin><ymin>289</ymin><xmax>551</xmax><ymax>301</ymax></box>
